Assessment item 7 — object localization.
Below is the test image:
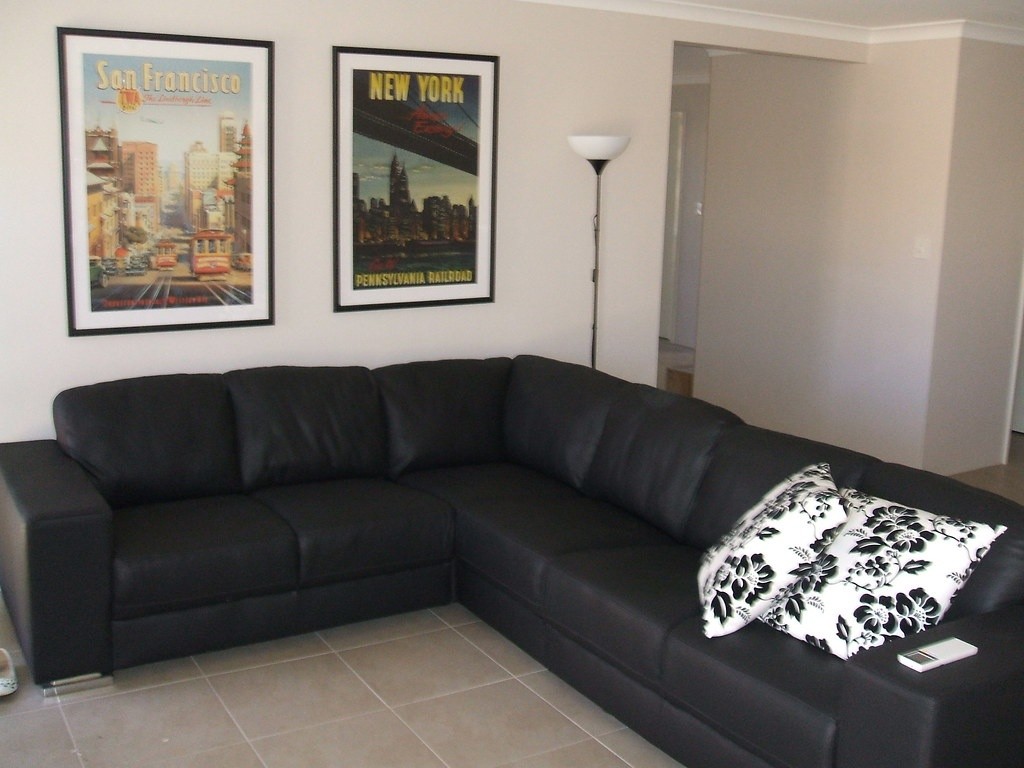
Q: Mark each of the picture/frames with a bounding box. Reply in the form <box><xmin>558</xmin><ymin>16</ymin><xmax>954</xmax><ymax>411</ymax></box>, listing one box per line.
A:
<box><xmin>57</xmin><ymin>25</ymin><xmax>274</xmax><ymax>336</ymax></box>
<box><xmin>332</xmin><ymin>45</ymin><xmax>500</xmax><ymax>311</ymax></box>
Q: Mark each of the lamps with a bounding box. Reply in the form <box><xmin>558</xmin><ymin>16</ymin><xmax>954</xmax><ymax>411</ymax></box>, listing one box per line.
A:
<box><xmin>567</xmin><ymin>134</ymin><xmax>633</xmax><ymax>370</ymax></box>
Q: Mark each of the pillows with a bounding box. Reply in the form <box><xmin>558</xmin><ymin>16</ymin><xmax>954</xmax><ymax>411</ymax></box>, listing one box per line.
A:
<box><xmin>757</xmin><ymin>488</ymin><xmax>1008</xmax><ymax>662</ymax></box>
<box><xmin>696</xmin><ymin>462</ymin><xmax>848</xmax><ymax>639</ymax></box>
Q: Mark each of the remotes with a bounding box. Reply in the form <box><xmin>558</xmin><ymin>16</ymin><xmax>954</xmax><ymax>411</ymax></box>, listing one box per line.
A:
<box><xmin>897</xmin><ymin>638</ymin><xmax>978</xmax><ymax>673</ymax></box>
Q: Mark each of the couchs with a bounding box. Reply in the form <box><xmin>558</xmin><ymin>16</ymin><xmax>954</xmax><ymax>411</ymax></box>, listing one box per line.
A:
<box><xmin>1</xmin><ymin>355</ymin><xmax>1024</xmax><ymax>768</ymax></box>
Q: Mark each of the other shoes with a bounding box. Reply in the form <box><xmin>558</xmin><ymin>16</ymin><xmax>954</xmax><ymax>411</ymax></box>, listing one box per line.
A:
<box><xmin>0</xmin><ymin>648</ymin><xmax>17</xmax><ymax>698</ymax></box>
